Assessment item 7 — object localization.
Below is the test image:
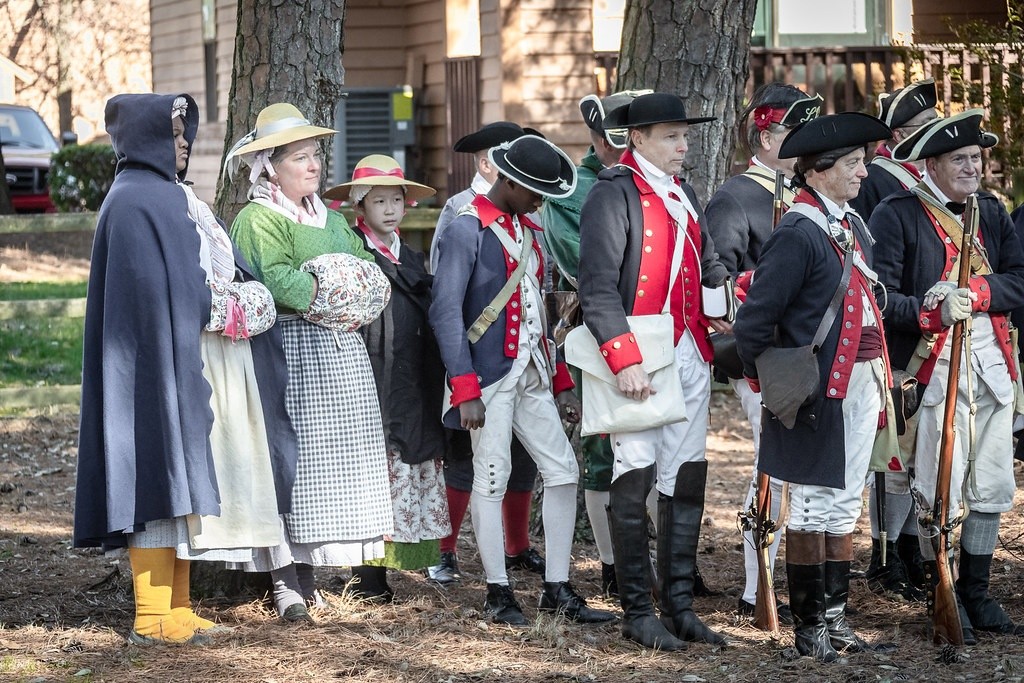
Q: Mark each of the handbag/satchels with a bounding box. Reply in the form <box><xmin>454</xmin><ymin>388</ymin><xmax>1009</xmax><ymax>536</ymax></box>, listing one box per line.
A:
<box><xmin>709</xmin><ymin>331</ymin><xmax>745</xmax><ymax>379</ymax></box>
<box><xmin>891</xmin><ymin>366</ymin><xmax>918</xmax><ymax>436</ymax></box>
<box><xmin>754</xmin><ymin>344</ymin><xmax>821</xmax><ymax>429</ymax></box>
<box><xmin>564</xmin><ymin>313</ymin><xmax>689</xmax><ymax>437</ymax></box>
<box><xmin>544</xmin><ymin>291</ymin><xmax>580</xmax><ymax>354</ymax></box>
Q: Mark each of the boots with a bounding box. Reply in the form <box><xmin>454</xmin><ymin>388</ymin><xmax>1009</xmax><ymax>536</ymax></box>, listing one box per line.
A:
<box><xmin>351</xmin><ymin>565</ymin><xmax>401</xmax><ymax>605</ymax></box>
<box><xmin>601</xmin><ymin>459</ymin><xmax>1024</xmax><ymax>665</ymax></box>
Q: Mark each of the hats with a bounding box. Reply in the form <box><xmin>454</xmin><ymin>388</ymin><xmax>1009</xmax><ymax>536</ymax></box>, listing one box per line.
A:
<box><xmin>891</xmin><ymin>108</ymin><xmax>999</xmax><ymax>163</ymax></box>
<box><xmin>453</xmin><ymin>121</ymin><xmax>546</xmax><ymax>153</ymax></box>
<box><xmin>222</xmin><ymin>102</ymin><xmax>341</xmax><ymax>185</ymax></box>
<box><xmin>878</xmin><ymin>77</ymin><xmax>937</xmax><ymax>130</ymax></box>
<box><xmin>487</xmin><ymin>135</ymin><xmax>577</xmax><ymax>198</ymax></box>
<box><xmin>322</xmin><ymin>154</ymin><xmax>437</xmax><ymax>205</ymax></box>
<box><xmin>602</xmin><ymin>92</ymin><xmax>718</xmax><ymax>129</ymax></box>
<box><xmin>778</xmin><ymin>111</ymin><xmax>894</xmax><ymax>159</ymax></box>
<box><xmin>578</xmin><ymin>89</ymin><xmax>654</xmax><ymax>149</ymax></box>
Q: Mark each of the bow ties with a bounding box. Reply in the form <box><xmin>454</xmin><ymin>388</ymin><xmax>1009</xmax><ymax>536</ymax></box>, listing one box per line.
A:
<box><xmin>945</xmin><ymin>202</ymin><xmax>966</xmax><ymax>215</ymax></box>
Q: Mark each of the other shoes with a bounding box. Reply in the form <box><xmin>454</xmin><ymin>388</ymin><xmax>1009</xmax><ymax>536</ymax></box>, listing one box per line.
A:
<box><xmin>128</xmin><ymin>600</ymin><xmax>368</xmax><ymax>647</ymax></box>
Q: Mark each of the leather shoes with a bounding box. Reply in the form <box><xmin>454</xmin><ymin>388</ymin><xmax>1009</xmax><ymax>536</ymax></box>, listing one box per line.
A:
<box><xmin>426</xmin><ymin>550</ymin><xmax>463</xmax><ymax>587</ymax></box>
<box><xmin>483</xmin><ymin>583</ymin><xmax>528</xmax><ymax>626</ymax></box>
<box><xmin>504</xmin><ymin>548</ymin><xmax>546</xmax><ymax>574</ymax></box>
<box><xmin>537</xmin><ymin>575</ymin><xmax>618</xmax><ymax>625</ymax></box>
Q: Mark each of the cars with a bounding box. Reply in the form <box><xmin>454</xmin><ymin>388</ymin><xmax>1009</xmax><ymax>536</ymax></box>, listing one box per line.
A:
<box><xmin>0</xmin><ymin>104</ymin><xmax>78</xmax><ymax>214</ymax></box>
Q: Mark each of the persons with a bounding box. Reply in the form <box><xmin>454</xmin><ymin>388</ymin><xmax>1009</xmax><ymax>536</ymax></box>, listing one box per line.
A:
<box><xmin>227</xmin><ymin>102</ymin><xmax>391</xmax><ymax>629</ymax></box>
<box><xmin>732</xmin><ymin>110</ymin><xmax>887</xmax><ymax>663</ymax></box>
<box><xmin>866</xmin><ymin>111</ymin><xmax>1024</xmax><ymax>642</ymax></box>
<box><xmin>579</xmin><ymin>94</ymin><xmax>746</xmax><ymax>650</ymax></box>
<box><xmin>429</xmin><ymin>137</ymin><xmax>617</xmax><ymax>627</ymax></box>
<box><xmin>321</xmin><ymin>78</ymin><xmax>937</xmax><ymax>607</ymax></box>
<box><xmin>72</xmin><ymin>94</ymin><xmax>277</xmax><ymax>648</ymax></box>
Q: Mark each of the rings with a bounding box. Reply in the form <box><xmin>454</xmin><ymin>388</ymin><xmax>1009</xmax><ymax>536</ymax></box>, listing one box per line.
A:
<box><xmin>721</xmin><ymin>331</ymin><xmax>727</xmax><ymax>336</ymax></box>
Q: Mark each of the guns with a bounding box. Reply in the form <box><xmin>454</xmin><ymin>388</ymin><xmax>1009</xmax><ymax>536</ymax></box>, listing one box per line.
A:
<box><xmin>921</xmin><ymin>194</ymin><xmax>974</xmax><ymax>648</ymax></box>
<box><xmin>739</xmin><ymin>168</ymin><xmax>785</xmax><ymax>632</ymax></box>
